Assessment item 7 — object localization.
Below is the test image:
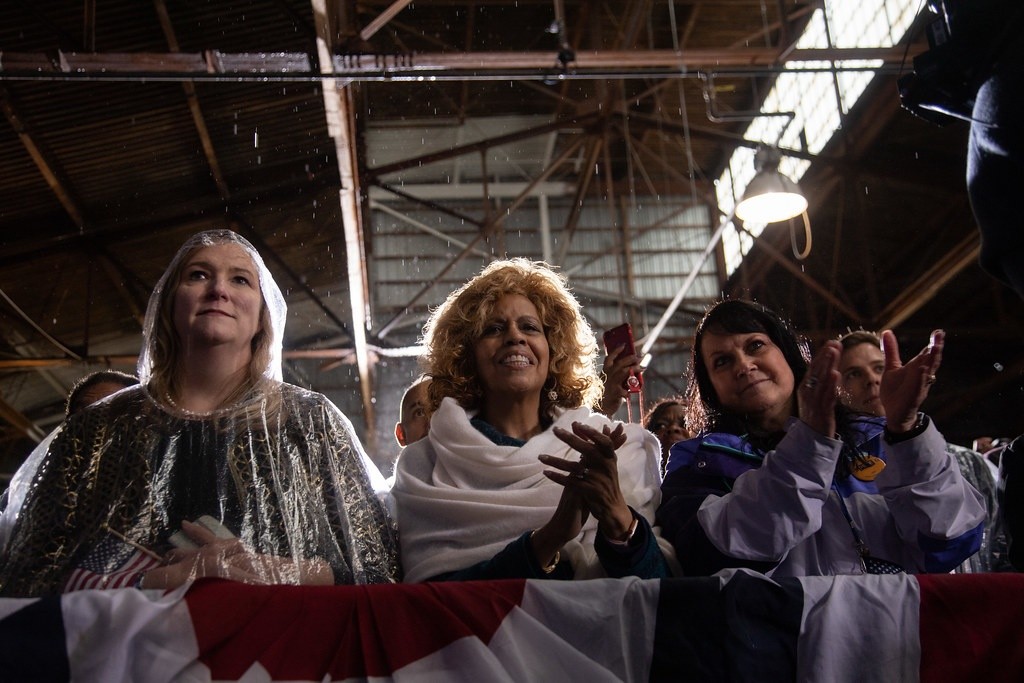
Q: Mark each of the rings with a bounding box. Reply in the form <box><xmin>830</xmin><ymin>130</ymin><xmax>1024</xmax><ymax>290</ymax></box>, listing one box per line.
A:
<box><xmin>927</xmin><ymin>374</ymin><xmax>936</xmax><ymax>384</ymax></box>
<box><xmin>583</xmin><ymin>463</ymin><xmax>592</xmax><ymax>474</ymax></box>
<box><xmin>569</xmin><ymin>471</ymin><xmax>586</xmax><ymax>481</ymax></box>
<box><xmin>804</xmin><ymin>383</ymin><xmax>815</xmax><ymax>390</ymax></box>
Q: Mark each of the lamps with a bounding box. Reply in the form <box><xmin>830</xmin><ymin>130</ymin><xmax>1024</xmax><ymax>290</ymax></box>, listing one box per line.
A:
<box><xmin>735</xmin><ymin>148</ymin><xmax>808</xmax><ymax>223</ymax></box>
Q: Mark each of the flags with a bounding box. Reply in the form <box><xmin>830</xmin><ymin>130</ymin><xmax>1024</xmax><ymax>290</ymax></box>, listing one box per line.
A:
<box><xmin>63</xmin><ymin>533</ymin><xmax>161</xmax><ymax>594</ymax></box>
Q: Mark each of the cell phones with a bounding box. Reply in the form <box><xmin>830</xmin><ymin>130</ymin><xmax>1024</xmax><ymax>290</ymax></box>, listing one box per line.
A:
<box><xmin>602</xmin><ymin>322</ymin><xmax>644</xmax><ymax>392</ymax></box>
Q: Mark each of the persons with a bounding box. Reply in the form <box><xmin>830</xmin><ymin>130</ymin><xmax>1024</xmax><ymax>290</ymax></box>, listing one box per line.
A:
<box><xmin>387</xmin><ymin>257</ymin><xmax>675</xmax><ymax>585</ymax></box>
<box><xmin>0</xmin><ymin>228</ymin><xmax>402</xmax><ymax>600</ymax></box>
<box><xmin>644</xmin><ymin>298</ymin><xmax>1024</xmax><ymax>578</ymax></box>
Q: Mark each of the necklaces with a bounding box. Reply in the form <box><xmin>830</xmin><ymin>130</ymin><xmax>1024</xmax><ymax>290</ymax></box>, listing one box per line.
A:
<box><xmin>163</xmin><ymin>389</ymin><xmax>234</xmax><ymax>417</ymax></box>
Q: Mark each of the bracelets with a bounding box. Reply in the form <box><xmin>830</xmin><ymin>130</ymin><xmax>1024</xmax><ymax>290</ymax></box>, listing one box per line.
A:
<box><xmin>134</xmin><ymin>570</ymin><xmax>146</xmax><ymax>590</ymax></box>
<box><xmin>531</xmin><ymin>529</ymin><xmax>560</xmax><ymax>574</ymax></box>
<box><xmin>601</xmin><ymin>518</ymin><xmax>639</xmax><ymax>546</ymax></box>
